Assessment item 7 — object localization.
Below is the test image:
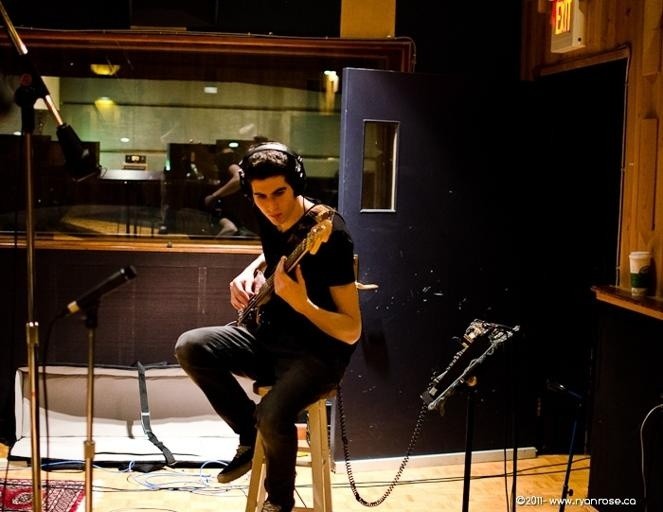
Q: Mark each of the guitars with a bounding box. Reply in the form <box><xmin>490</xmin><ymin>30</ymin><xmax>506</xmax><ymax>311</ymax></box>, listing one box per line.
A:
<box><xmin>235</xmin><ymin>219</ymin><xmax>334</xmax><ymax>333</ymax></box>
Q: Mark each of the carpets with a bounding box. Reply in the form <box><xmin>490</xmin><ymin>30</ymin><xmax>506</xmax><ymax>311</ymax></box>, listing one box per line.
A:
<box><xmin>3</xmin><ymin>477</ymin><xmax>86</xmax><ymax>512</ymax></box>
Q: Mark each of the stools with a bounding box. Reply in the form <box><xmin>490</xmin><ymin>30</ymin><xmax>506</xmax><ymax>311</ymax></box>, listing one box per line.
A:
<box><xmin>245</xmin><ymin>381</ymin><xmax>339</xmax><ymax>512</ymax></box>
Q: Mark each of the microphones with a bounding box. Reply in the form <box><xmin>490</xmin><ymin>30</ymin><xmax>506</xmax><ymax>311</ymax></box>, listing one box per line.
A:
<box><xmin>60</xmin><ymin>264</ymin><xmax>138</xmax><ymax>318</ymax></box>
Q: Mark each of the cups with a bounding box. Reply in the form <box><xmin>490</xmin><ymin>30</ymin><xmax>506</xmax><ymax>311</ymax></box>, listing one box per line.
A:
<box><xmin>629</xmin><ymin>251</ymin><xmax>652</xmax><ymax>297</ymax></box>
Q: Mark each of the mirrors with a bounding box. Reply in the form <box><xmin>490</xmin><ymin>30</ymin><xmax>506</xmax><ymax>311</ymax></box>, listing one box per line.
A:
<box><xmin>2</xmin><ymin>22</ymin><xmax>414</xmax><ymax>254</ymax></box>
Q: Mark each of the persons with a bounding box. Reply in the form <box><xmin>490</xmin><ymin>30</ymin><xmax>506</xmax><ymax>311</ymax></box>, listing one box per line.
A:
<box><xmin>204</xmin><ymin>147</ymin><xmax>246</xmax><ymax>237</ymax></box>
<box><xmin>174</xmin><ymin>141</ymin><xmax>362</xmax><ymax>512</ymax></box>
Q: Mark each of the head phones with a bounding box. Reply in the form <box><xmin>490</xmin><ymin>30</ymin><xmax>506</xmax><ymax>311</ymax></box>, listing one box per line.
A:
<box><xmin>239</xmin><ymin>144</ymin><xmax>307</xmax><ymax>200</ymax></box>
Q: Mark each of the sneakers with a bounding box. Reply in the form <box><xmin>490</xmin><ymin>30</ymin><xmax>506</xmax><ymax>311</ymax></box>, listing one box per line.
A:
<box><xmin>261</xmin><ymin>497</ymin><xmax>294</xmax><ymax>512</ymax></box>
<box><xmin>218</xmin><ymin>444</ymin><xmax>254</xmax><ymax>483</ymax></box>
